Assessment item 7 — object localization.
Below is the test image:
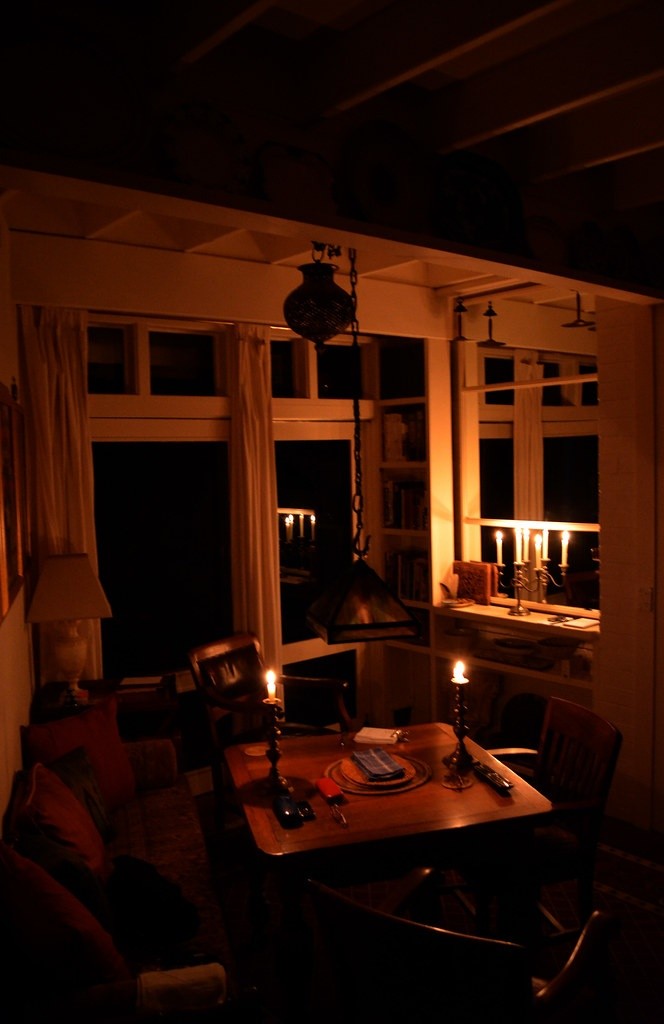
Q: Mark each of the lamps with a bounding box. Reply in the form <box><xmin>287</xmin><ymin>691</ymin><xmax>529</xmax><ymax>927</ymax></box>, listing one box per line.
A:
<box><xmin>283</xmin><ymin>239</ymin><xmax>355</xmax><ymax>348</ymax></box>
<box><xmin>304</xmin><ymin>249</ymin><xmax>424</xmax><ymax>643</ymax></box>
<box><xmin>25</xmin><ymin>552</ymin><xmax>114</xmax><ymax>704</ymax></box>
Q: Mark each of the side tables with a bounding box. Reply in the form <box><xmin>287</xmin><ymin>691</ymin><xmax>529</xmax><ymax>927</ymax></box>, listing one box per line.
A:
<box><xmin>31</xmin><ymin>679</ymin><xmax>118</xmax><ymax>723</ymax></box>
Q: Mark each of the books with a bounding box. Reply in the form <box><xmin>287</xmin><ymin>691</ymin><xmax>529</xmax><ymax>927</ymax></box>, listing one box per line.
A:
<box><xmin>383</xmin><ymin>479</ymin><xmax>427</xmax><ymax>529</ymax></box>
<box><xmin>385</xmin><ymin>551</ymin><xmax>428</xmax><ymax>601</ymax></box>
<box><xmin>354</xmin><ymin>727</ymin><xmax>413</xmax><ymax>743</ymax></box>
<box><xmin>454</xmin><ymin>562</ymin><xmax>491</xmax><ymax>604</ymax></box>
<box><xmin>383</xmin><ymin>409</ymin><xmax>425</xmax><ymax>461</ymax></box>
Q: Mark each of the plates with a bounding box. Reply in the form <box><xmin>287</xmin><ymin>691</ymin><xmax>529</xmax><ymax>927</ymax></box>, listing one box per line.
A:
<box><xmin>449</xmin><ymin>597</ymin><xmax>475</xmax><ymax>608</ymax></box>
<box><xmin>324</xmin><ymin>753</ymin><xmax>433</xmax><ymax>795</ymax></box>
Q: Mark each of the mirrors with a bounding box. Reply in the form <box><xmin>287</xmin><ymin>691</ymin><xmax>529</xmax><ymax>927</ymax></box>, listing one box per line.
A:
<box><xmin>444</xmin><ymin>282</ymin><xmax>601</xmax><ymax>612</ymax></box>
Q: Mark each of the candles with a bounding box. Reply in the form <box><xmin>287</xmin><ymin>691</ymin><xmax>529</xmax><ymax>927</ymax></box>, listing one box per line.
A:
<box><xmin>495</xmin><ymin>531</ymin><xmax>504</xmax><ymax>564</ymax></box>
<box><xmin>534</xmin><ymin>533</ymin><xmax>542</xmax><ymax>567</ymax></box>
<box><xmin>284</xmin><ymin>513</ymin><xmax>316</xmax><ymax>543</ymax></box>
<box><xmin>516</xmin><ymin>525</ymin><xmax>522</xmax><ymax>561</ymax></box>
<box><xmin>452</xmin><ymin>662</ymin><xmax>467</xmax><ymax>684</ymax></box>
<box><xmin>265</xmin><ymin>670</ymin><xmax>276</xmax><ymax>702</ymax></box>
<box><xmin>521</xmin><ymin>527</ymin><xmax>570</xmax><ymax>564</ymax></box>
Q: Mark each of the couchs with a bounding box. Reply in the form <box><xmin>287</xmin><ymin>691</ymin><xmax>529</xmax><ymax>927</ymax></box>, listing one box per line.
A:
<box><xmin>0</xmin><ymin>738</ymin><xmax>241</xmax><ymax>1024</ymax></box>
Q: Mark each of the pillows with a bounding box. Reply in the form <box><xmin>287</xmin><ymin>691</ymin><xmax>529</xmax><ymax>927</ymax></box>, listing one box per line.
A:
<box><xmin>20</xmin><ymin>694</ymin><xmax>136</xmax><ymax>812</ymax></box>
<box><xmin>13</xmin><ymin>762</ymin><xmax>104</xmax><ymax>881</ymax></box>
<box><xmin>49</xmin><ymin>746</ymin><xmax>117</xmax><ymax>843</ymax></box>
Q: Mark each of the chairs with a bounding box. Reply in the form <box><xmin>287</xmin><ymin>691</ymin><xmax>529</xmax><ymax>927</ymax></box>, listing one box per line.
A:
<box><xmin>295</xmin><ymin>874</ymin><xmax>606</xmax><ymax>1024</ymax></box>
<box><xmin>438</xmin><ymin>688</ymin><xmax>623</xmax><ymax>939</ymax></box>
<box><xmin>182</xmin><ymin>631</ymin><xmax>351</xmax><ymax>838</ymax></box>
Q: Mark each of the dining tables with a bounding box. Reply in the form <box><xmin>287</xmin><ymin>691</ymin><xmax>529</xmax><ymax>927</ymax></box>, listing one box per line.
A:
<box><xmin>223</xmin><ymin>718</ymin><xmax>558</xmax><ymax>1010</ymax></box>
